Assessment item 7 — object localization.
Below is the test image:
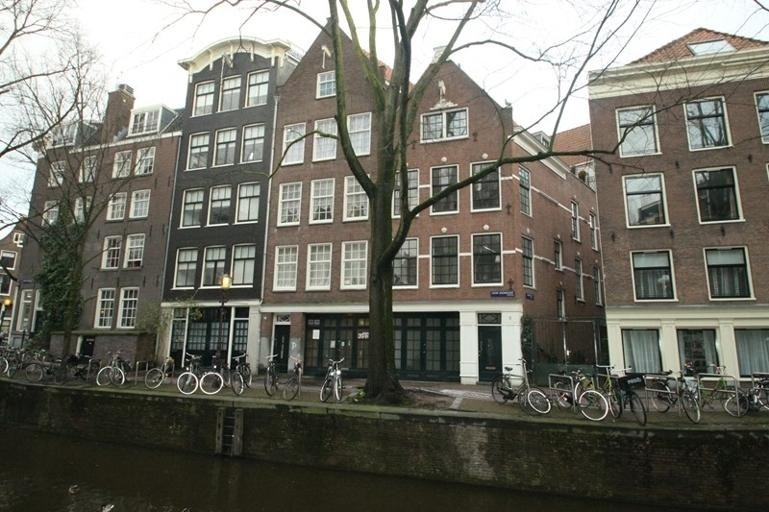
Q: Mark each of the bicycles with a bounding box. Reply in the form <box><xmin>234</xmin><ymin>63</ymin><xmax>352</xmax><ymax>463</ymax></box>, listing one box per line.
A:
<box><xmin>263</xmin><ymin>354</ymin><xmax>282</xmax><ymax>395</ymax></box>
<box><xmin>176</xmin><ymin>352</ymin><xmax>200</xmax><ymax>395</ymax></box>
<box><xmin>491</xmin><ymin>358</ymin><xmax>544</xmax><ymax>419</ymax></box>
<box><xmin>144</xmin><ymin>357</ymin><xmax>175</xmax><ymax>390</ymax></box>
<box><xmin>281</xmin><ymin>355</ymin><xmax>305</xmax><ymax>401</ymax></box>
<box><xmin>230</xmin><ymin>353</ymin><xmax>253</xmax><ymax>394</ymax></box>
<box><xmin>318</xmin><ymin>357</ymin><xmax>345</xmax><ymax>403</ymax></box>
<box><xmin>525</xmin><ymin>363</ymin><xmax>768</xmax><ymax>426</ymax></box>
<box><xmin>199</xmin><ymin>354</ymin><xmax>232</xmax><ymax>395</ymax></box>
<box><xmin>0</xmin><ymin>345</ymin><xmax>130</xmax><ymax>388</ymax></box>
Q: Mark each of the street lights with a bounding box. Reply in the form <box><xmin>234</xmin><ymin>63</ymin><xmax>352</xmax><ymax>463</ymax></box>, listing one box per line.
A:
<box><xmin>215</xmin><ymin>273</ymin><xmax>233</xmax><ymax>389</ymax></box>
<box><xmin>0</xmin><ymin>299</ymin><xmax>12</xmax><ymax>327</ymax></box>
